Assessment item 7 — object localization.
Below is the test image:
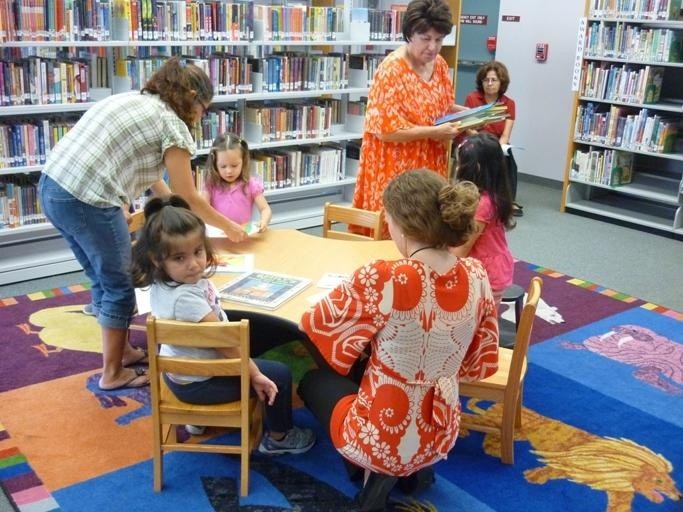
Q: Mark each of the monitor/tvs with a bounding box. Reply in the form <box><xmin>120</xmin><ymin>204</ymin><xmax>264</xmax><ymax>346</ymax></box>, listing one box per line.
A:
<box><xmin>215</xmin><ymin>268</ymin><xmax>311</xmax><ymax>311</ymax></box>
<box><xmin>117</xmin><ymin>49</ymin><xmax>346</xmax><ymax>91</ymax></box>
<box><xmin>349</xmin><ymin>52</ymin><xmax>388</xmax><ymax>88</ymax></box>
<box><xmin>194</xmin><ymin>145</ymin><xmax>342</xmax><ymax>191</ymax></box>
<box><xmin>435</xmin><ymin>102</ymin><xmax>511</xmax><ymax>134</ymax></box>
<box><xmin>0</xmin><ymin>119</ymin><xmax>78</xmax><ymax>168</ymax></box>
<box><xmin>350</xmin><ymin>4</ymin><xmax>409</xmax><ymax>43</ymax></box>
<box><xmin>192</xmin><ymin>98</ymin><xmax>368</xmax><ymax>151</ymax></box>
<box><xmin>0</xmin><ymin>179</ymin><xmax>51</xmax><ymax>228</ymax></box>
<box><xmin>569</xmin><ymin>1</ymin><xmax>682</xmax><ymax>189</ymax></box>
<box><xmin>0</xmin><ymin>52</ymin><xmax>110</xmax><ymax>108</ymax></box>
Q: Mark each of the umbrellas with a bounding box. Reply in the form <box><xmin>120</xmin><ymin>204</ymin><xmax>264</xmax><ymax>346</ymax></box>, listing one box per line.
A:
<box><xmin>511</xmin><ymin>202</ymin><xmax>523</xmax><ymax>216</ymax></box>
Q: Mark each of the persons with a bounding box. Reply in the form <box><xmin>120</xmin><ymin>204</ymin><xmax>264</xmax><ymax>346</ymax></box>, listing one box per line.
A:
<box><xmin>296</xmin><ymin>166</ymin><xmax>500</xmax><ymax>511</ymax></box>
<box><xmin>348</xmin><ymin>0</ymin><xmax>472</xmax><ymax>240</ymax></box>
<box><xmin>199</xmin><ymin>132</ymin><xmax>273</xmax><ymax>235</ymax></box>
<box><xmin>125</xmin><ymin>193</ymin><xmax>316</xmax><ymax>459</ymax></box>
<box><xmin>37</xmin><ymin>56</ymin><xmax>250</xmax><ymax>389</ymax></box>
<box><xmin>462</xmin><ymin>61</ymin><xmax>523</xmax><ymax>217</ymax></box>
<box><xmin>450</xmin><ymin>131</ymin><xmax>522</xmax><ymax>301</ymax></box>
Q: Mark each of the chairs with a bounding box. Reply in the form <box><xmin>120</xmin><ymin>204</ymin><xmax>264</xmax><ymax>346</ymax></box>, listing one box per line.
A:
<box><xmin>257</xmin><ymin>426</ymin><xmax>318</xmax><ymax>456</ymax></box>
<box><xmin>401</xmin><ymin>464</ymin><xmax>435</xmax><ymax>497</ymax></box>
<box><xmin>355</xmin><ymin>470</ymin><xmax>398</xmax><ymax>512</ymax></box>
<box><xmin>185</xmin><ymin>425</ymin><xmax>207</xmax><ymax>436</ymax></box>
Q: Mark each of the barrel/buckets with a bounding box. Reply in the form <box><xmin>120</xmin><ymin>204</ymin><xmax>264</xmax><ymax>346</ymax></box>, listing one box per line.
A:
<box><xmin>98</xmin><ymin>367</ymin><xmax>150</xmax><ymax>390</ymax></box>
<box><xmin>123</xmin><ymin>349</ymin><xmax>150</xmax><ymax>367</ymax></box>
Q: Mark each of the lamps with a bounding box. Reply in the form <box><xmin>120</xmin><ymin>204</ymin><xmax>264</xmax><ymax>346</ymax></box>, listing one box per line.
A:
<box><xmin>129</xmin><ymin>210</ymin><xmax>147</xmax><ymax>246</ymax></box>
<box><xmin>459</xmin><ymin>277</ymin><xmax>543</xmax><ymax>464</ymax></box>
<box><xmin>323</xmin><ymin>202</ymin><xmax>384</xmax><ymax>241</ymax></box>
<box><xmin>146</xmin><ymin>315</ymin><xmax>263</xmax><ymax>496</ymax></box>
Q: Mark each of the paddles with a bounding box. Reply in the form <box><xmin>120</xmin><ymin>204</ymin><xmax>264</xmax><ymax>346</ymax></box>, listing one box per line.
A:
<box><xmin>1</xmin><ymin>256</ymin><xmax>683</xmax><ymax>512</ymax></box>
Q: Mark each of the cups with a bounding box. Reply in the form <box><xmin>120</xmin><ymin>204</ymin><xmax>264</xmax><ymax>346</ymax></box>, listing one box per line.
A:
<box><xmin>197</xmin><ymin>98</ymin><xmax>209</xmax><ymax>119</ymax></box>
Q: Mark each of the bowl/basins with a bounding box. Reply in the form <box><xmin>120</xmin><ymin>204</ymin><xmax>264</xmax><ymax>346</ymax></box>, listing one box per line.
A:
<box><xmin>409</xmin><ymin>246</ymin><xmax>437</xmax><ymax>258</ymax></box>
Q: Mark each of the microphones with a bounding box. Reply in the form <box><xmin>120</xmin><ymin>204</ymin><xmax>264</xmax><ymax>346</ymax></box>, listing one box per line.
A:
<box><xmin>502</xmin><ymin>283</ymin><xmax>525</xmax><ymax>331</ymax></box>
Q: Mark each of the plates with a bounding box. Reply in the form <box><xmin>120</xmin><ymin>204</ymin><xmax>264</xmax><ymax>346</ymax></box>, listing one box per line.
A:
<box><xmin>1</xmin><ymin>0</ymin><xmax>462</xmax><ymax>286</ymax></box>
<box><xmin>560</xmin><ymin>0</ymin><xmax>683</xmax><ymax>235</ymax></box>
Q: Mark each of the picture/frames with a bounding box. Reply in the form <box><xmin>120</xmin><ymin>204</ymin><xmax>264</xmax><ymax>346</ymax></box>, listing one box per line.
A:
<box><xmin>205</xmin><ymin>227</ymin><xmax>407</xmax><ymax>382</ymax></box>
